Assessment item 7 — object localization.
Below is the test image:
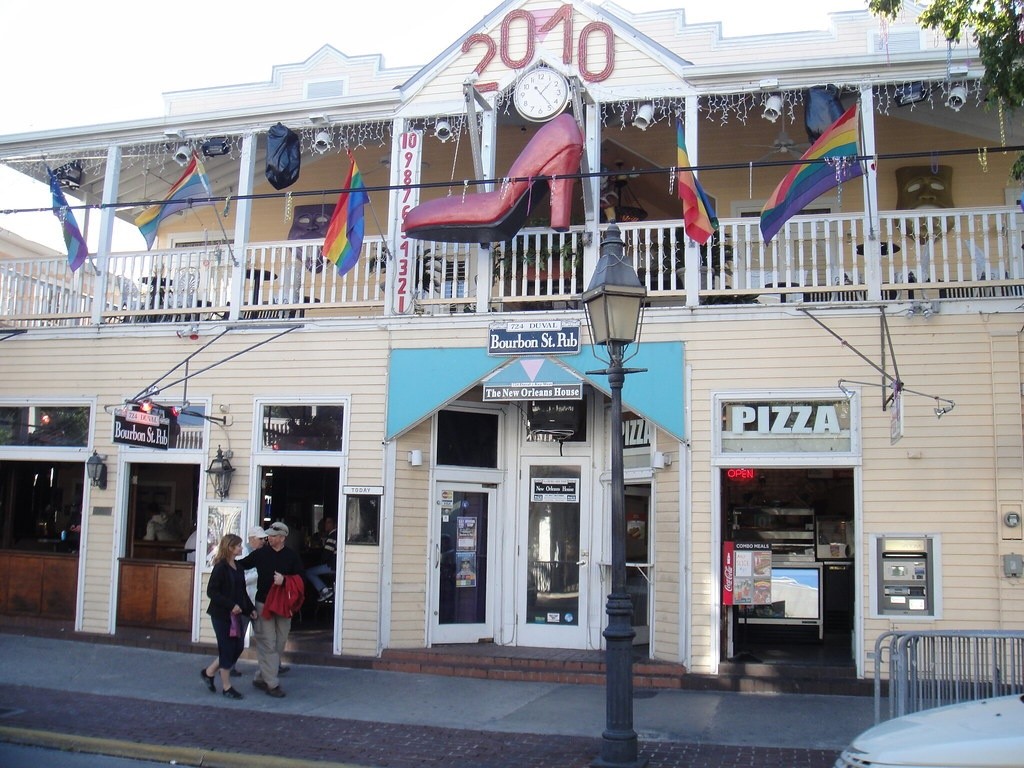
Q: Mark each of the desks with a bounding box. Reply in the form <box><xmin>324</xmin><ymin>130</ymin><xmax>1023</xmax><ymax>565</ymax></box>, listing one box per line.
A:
<box><xmin>857</xmin><ymin>242</ymin><xmax>900</xmax><ymax>256</ymax></box>
<box><xmin>245</xmin><ymin>268</ymin><xmax>279</xmax><ymax>320</ymax></box>
<box><xmin>39</xmin><ymin>538</ymin><xmax>73</xmax><ymax>551</ymax></box>
<box><xmin>763</xmin><ymin>281</ymin><xmax>800</xmax><ymax>303</ymax></box>
<box><xmin>128</xmin><ymin>540</ymin><xmax>190</xmax><ymax>562</ymax></box>
<box><xmin>140</xmin><ymin>277</ymin><xmax>172</xmax><ymax>323</ymax></box>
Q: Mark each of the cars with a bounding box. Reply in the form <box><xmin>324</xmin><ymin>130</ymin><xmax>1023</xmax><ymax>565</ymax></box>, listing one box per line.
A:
<box><xmin>832</xmin><ymin>692</ymin><xmax>1024</xmax><ymax>768</ymax></box>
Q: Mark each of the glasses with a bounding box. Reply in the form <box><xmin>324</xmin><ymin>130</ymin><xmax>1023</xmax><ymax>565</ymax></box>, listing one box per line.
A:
<box><xmin>269</xmin><ymin>525</ymin><xmax>287</xmax><ymax>535</ymax></box>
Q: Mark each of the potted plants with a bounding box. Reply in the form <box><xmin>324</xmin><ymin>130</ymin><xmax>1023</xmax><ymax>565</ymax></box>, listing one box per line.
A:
<box><xmin>655</xmin><ymin>227</ymin><xmax>736</xmax><ymax>291</ymax></box>
<box><xmin>483</xmin><ymin>220</ymin><xmax>584</xmax><ymax>284</ymax></box>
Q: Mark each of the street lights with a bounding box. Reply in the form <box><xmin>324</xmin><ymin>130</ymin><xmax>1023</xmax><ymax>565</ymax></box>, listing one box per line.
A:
<box><xmin>579</xmin><ymin>216</ymin><xmax>651</xmax><ymax>768</ymax></box>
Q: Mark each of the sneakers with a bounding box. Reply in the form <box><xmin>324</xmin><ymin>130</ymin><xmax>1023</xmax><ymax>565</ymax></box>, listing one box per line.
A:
<box><xmin>317</xmin><ymin>589</ymin><xmax>334</xmax><ymax>602</ymax></box>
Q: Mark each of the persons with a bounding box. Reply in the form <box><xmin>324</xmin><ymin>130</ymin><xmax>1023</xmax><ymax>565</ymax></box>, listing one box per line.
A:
<box><xmin>201</xmin><ymin>534</ymin><xmax>257</xmax><ymax>699</ymax></box>
<box><xmin>304</xmin><ymin>513</ymin><xmax>337</xmax><ymax>602</ymax></box>
<box><xmin>238</xmin><ymin>522</ymin><xmax>303</xmax><ymax>698</ymax></box>
<box><xmin>219</xmin><ymin>526</ymin><xmax>290</xmax><ymax>677</ymax></box>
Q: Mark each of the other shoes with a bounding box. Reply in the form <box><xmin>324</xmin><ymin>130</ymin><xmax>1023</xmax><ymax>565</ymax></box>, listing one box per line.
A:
<box><xmin>266</xmin><ymin>686</ymin><xmax>285</xmax><ymax>698</ymax></box>
<box><xmin>253</xmin><ymin>679</ymin><xmax>268</xmax><ymax>690</ymax></box>
<box><xmin>278</xmin><ymin>666</ymin><xmax>290</xmax><ymax>675</ymax></box>
<box><xmin>222</xmin><ymin>688</ymin><xmax>243</xmax><ymax>700</ymax></box>
<box><xmin>219</xmin><ymin>669</ymin><xmax>242</xmax><ymax>677</ymax></box>
<box><xmin>200</xmin><ymin>668</ymin><xmax>216</xmax><ymax>692</ymax></box>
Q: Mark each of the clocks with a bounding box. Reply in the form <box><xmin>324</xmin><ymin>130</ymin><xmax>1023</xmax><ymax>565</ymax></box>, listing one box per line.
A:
<box><xmin>512</xmin><ymin>68</ymin><xmax>570</xmax><ymax>123</ymax></box>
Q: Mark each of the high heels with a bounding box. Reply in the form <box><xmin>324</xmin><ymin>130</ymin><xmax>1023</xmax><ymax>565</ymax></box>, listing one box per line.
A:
<box><xmin>404</xmin><ymin>113</ymin><xmax>583</xmax><ymax>244</ymax></box>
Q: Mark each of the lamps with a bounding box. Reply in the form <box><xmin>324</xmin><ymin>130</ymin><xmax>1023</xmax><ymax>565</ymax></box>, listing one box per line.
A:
<box><xmin>652</xmin><ymin>450</ymin><xmax>672</xmax><ymax>468</ymax></box>
<box><xmin>53</xmin><ymin>161</ymin><xmax>83</xmax><ymax>191</ymax></box>
<box><xmin>796</xmin><ymin>299</ymin><xmax>956</xmax><ymax>420</ymax></box>
<box><xmin>603</xmin><ymin>157</ymin><xmax>649</xmax><ymax>223</ymax></box>
<box><xmin>164</xmin><ymin>128</ymin><xmax>228</xmax><ymax>166</ymax></box>
<box><xmin>893</xmin><ymin>66</ymin><xmax>969</xmax><ymax>111</ymax></box>
<box><xmin>760</xmin><ymin>78</ymin><xmax>782</xmax><ymax>123</ymax></box>
<box><xmin>309</xmin><ymin>112</ymin><xmax>331</xmax><ymax>154</ymax></box>
<box><xmin>631</xmin><ymin>104</ymin><xmax>654</xmax><ymax>131</ymax></box>
<box><xmin>407</xmin><ymin>450</ymin><xmax>422</xmax><ymax>466</ymax></box>
<box><xmin>86</xmin><ymin>449</ymin><xmax>107</xmax><ymax>490</ymax></box>
<box><xmin>204</xmin><ymin>445</ymin><xmax>237</xmax><ymax>499</ymax></box>
<box><xmin>102</xmin><ymin>324</ymin><xmax>305</xmax><ymax>425</ymax></box>
<box><xmin>434</xmin><ymin>121</ymin><xmax>454</xmax><ymax>144</ymax></box>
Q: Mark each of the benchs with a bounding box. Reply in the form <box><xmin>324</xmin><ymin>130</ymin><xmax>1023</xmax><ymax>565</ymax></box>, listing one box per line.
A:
<box><xmin>119</xmin><ymin>297</ymin><xmax>321</xmax><ymax>324</ymax></box>
<box><xmin>803</xmin><ymin>276</ymin><xmax>1024</xmax><ymax>301</ymax></box>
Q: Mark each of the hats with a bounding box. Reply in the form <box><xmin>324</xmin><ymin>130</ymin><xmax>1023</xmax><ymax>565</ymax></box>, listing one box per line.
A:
<box><xmin>248</xmin><ymin>526</ymin><xmax>268</xmax><ymax>538</ymax></box>
<box><xmin>265</xmin><ymin>521</ymin><xmax>289</xmax><ymax>536</ymax></box>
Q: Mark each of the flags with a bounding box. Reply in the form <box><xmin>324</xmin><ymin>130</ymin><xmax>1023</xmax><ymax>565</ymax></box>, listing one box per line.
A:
<box><xmin>677</xmin><ymin>116</ymin><xmax>720</xmax><ymax>246</ymax></box>
<box><xmin>135</xmin><ymin>148</ymin><xmax>216</xmax><ymax>252</ymax></box>
<box><xmin>45</xmin><ymin>165</ymin><xmax>89</xmax><ymax>272</ymax></box>
<box><xmin>760</xmin><ymin>96</ymin><xmax>868</xmax><ymax>246</ymax></box>
<box><xmin>322</xmin><ymin>146</ymin><xmax>370</xmax><ymax>277</ymax></box>
